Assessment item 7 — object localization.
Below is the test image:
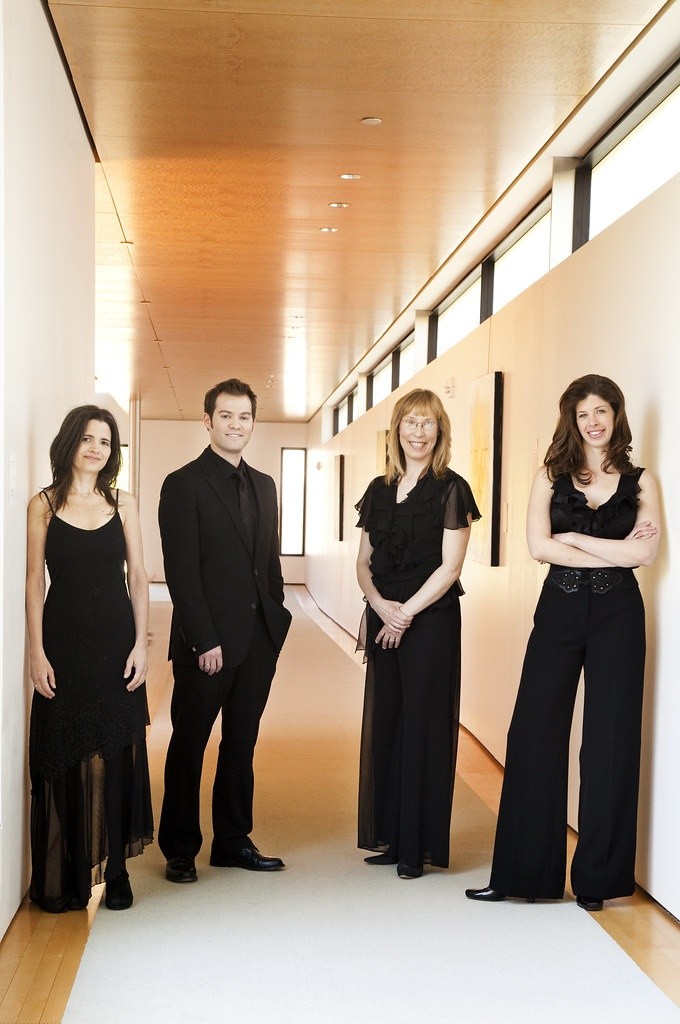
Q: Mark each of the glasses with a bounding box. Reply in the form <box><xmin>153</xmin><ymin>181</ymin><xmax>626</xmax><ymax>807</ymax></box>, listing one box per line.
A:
<box><xmin>401</xmin><ymin>419</ymin><xmax>438</xmax><ymax>430</ymax></box>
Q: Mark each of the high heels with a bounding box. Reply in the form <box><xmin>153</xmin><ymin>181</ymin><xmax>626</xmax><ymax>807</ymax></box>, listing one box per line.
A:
<box><xmin>466</xmin><ymin>886</ymin><xmax>536</xmax><ymax>904</ymax></box>
<box><xmin>574</xmin><ymin>894</ymin><xmax>605</xmax><ymax>912</ymax></box>
<box><xmin>363</xmin><ymin>842</ymin><xmax>403</xmax><ymax>865</ymax></box>
<box><xmin>397</xmin><ymin>845</ymin><xmax>424</xmax><ymax>878</ymax></box>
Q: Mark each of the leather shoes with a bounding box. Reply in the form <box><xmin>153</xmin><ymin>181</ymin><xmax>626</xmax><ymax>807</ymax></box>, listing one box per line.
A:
<box><xmin>166</xmin><ymin>857</ymin><xmax>198</xmax><ymax>881</ymax></box>
<box><xmin>210</xmin><ymin>837</ymin><xmax>285</xmax><ymax>870</ymax></box>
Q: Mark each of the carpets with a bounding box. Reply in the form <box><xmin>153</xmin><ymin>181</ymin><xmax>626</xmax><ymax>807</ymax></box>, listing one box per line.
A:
<box><xmin>58</xmin><ymin>734</ymin><xmax>679</xmax><ymax>1024</ymax></box>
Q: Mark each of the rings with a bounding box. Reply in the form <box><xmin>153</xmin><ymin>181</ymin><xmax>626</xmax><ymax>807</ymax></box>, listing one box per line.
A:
<box><xmin>34</xmin><ymin>685</ymin><xmax>38</xmax><ymax>688</ymax></box>
<box><xmin>205</xmin><ymin>663</ymin><xmax>210</xmax><ymax>666</ymax></box>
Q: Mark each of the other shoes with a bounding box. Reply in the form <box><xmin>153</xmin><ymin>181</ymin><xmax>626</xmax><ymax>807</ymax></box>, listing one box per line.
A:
<box><xmin>103</xmin><ymin>862</ymin><xmax>133</xmax><ymax>909</ymax></box>
<box><xmin>63</xmin><ymin>867</ymin><xmax>91</xmax><ymax>910</ymax></box>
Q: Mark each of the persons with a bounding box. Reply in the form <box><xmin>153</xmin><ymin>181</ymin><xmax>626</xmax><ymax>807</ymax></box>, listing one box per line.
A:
<box><xmin>463</xmin><ymin>372</ymin><xmax>663</xmax><ymax>912</ymax></box>
<box><xmin>159</xmin><ymin>378</ymin><xmax>293</xmax><ymax>884</ymax></box>
<box><xmin>351</xmin><ymin>389</ymin><xmax>482</xmax><ymax>882</ymax></box>
<box><xmin>26</xmin><ymin>402</ymin><xmax>155</xmax><ymax>912</ymax></box>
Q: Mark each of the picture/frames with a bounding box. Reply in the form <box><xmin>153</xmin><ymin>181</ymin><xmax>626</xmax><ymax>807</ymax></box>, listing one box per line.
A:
<box><xmin>469</xmin><ymin>370</ymin><xmax>502</xmax><ymax>567</ymax></box>
<box><xmin>376</xmin><ymin>430</ymin><xmax>390</xmax><ymax>478</ymax></box>
<box><xmin>334</xmin><ymin>455</ymin><xmax>344</xmax><ymax>541</ymax></box>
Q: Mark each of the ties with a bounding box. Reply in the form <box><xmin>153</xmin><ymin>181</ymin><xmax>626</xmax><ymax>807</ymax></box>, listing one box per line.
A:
<box><xmin>236</xmin><ymin>469</ymin><xmax>256</xmax><ymax>558</ymax></box>
